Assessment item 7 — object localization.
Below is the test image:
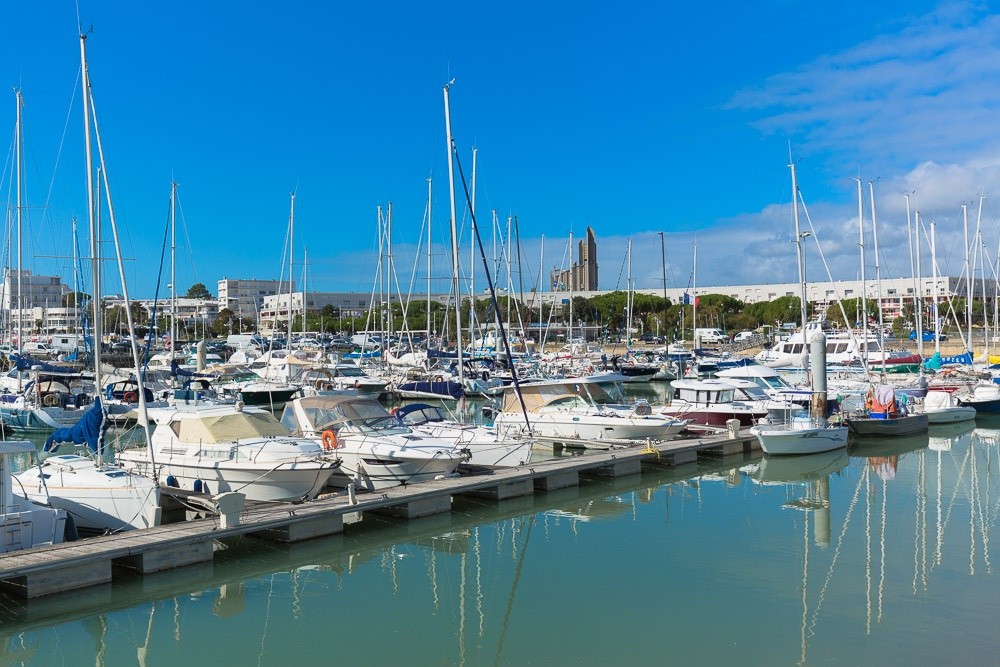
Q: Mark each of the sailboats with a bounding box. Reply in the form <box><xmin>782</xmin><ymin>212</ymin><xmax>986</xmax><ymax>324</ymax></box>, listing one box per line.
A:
<box><xmin>924</xmin><ymin>189</ymin><xmax>975</xmax><ymax>422</ymax></box>
<box><xmin>749</xmin><ymin>162</ymin><xmax>852</xmax><ymax>455</ymax></box>
<box><xmin>963</xmin><ymin>196</ymin><xmax>1000</xmax><ymax>413</ymax></box>
<box><xmin>953</xmin><ymin>415</ymin><xmax>1000</xmax><ymax>575</ymax></box>
<box><xmin>846</xmin><ymin>177</ymin><xmax>931</xmax><ymax>438</ymax></box>
<box><xmin>849</xmin><ymin>433</ymin><xmax>931</xmax><ymax>635</ymax></box>
<box><xmin>0</xmin><ymin>31</ymin><xmax>900</xmax><ymax>551</ymax></box>
<box><xmin>750</xmin><ymin>449</ymin><xmax>849</xmax><ymax>661</ymax></box>
<box><xmin>925</xmin><ymin>420</ymin><xmax>978</xmax><ymax>568</ymax></box>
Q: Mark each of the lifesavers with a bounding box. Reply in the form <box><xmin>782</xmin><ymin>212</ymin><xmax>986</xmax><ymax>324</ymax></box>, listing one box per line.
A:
<box><xmin>436</xmin><ymin>376</ymin><xmax>443</xmax><ymax>383</ymax></box>
<box><xmin>44</xmin><ymin>395</ymin><xmax>57</xmax><ymax>405</ymax></box>
<box><xmin>826</xmin><ymin>407</ymin><xmax>832</xmax><ymax>416</ymax></box>
<box><xmin>124</xmin><ymin>392</ymin><xmax>137</xmax><ymax>401</ymax></box>
<box><xmin>629</xmin><ymin>361</ymin><xmax>634</xmax><ymax>366</ymax></box>
<box><xmin>390</xmin><ymin>408</ymin><xmax>398</xmax><ymax>415</ymax></box>
<box><xmin>322</xmin><ymin>430</ymin><xmax>336</xmax><ymax>448</ymax></box>
<box><xmin>317</xmin><ymin>381</ymin><xmax>324</xmax><ymax>388</ymax></box>
<box><xmin>76</xmin><ymin>393</ymin><xmax>90</xmax><ymax>409</ymax></box>
